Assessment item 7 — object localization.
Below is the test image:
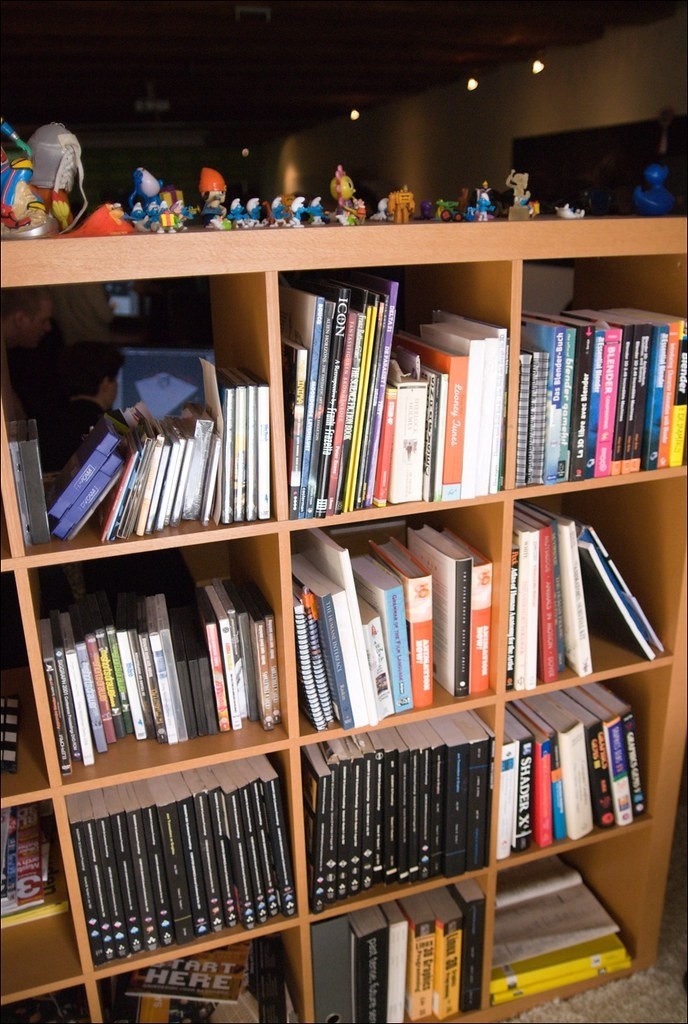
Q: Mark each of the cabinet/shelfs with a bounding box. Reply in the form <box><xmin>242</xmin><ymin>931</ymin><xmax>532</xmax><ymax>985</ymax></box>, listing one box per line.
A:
<box><xmin>0</xmin><ymin>217</ymin><xmax>688</xmax><ymax>1024</ymax></box>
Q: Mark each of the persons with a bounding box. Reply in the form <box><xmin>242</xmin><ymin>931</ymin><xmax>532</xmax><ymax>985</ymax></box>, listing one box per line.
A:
<box><xmin>123</xmin><ymin>200</ymin><xmax>188</xmax><ymax>234</ymax></box>
<box><xmin>226</xmin><ymin>199</ymin><xmax>249</xmax><ymax>229</ymax></box>
<box><xmin>269</xmin><ymin>196</ymin><xmax>292</xmax><ymax>228</ymax></box>
<box><xmin>477</xmin><ymin>193</ymin><xmax>492</xmax><ymax>222</ymax></box>
<box><xmin>243</xmin><ymin>197</ymin><xmax>265</xmax><ymax>229</ymax></box>
<box><xmin>286</xmin><ymin>197</ymin><xmax>309</xmax><ymax>228</ymax></box>
<box><xmin>199</xmin><ymin>168</ymin><xmax>227</xmax><ymax>228</ymax></box>
<box><xmin>304</xmin><ymin>197</ymin><xmax>331</xmax><ymax>226</ymax></box>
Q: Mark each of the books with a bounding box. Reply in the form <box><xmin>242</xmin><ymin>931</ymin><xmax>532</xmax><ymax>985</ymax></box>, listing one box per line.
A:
<box><xmin>1</xmin><ymin>270</ymin><xmax>687</xmax><ymax>1024</ymax></box>
